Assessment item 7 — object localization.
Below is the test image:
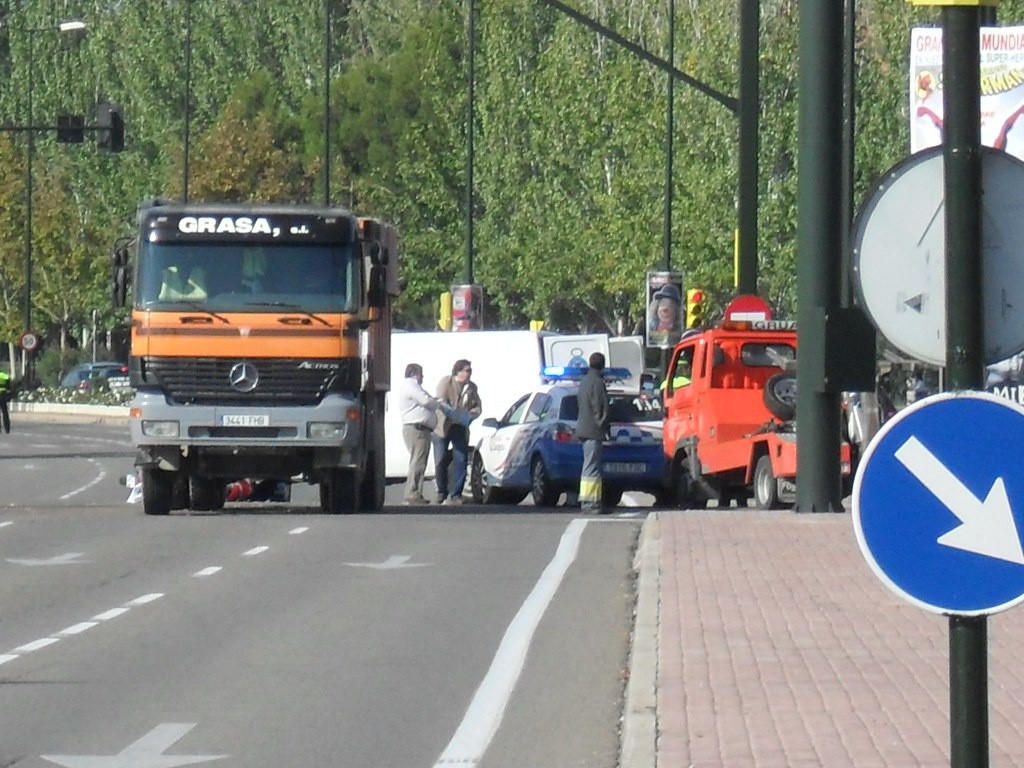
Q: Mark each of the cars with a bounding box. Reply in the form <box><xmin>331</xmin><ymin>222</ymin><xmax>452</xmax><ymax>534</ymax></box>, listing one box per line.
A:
<box><xmin>57</xmin><ymin>356</ymin><xmax>126</xmax><ymax>393</ymax></box>
<box><xmin>469</xmin><ymin>379</ymin><xmax>668</xmax><ymax>507</ymax></box>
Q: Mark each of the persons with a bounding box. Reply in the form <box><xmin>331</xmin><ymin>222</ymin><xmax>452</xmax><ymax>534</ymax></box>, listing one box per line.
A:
<box><xmin>397</xmin><ymin>364</ymin><xmax>442</xmax><ymax>505</ymax></box>
<box><xmin>574</xmin><ymin>351</ymin><xmax>612</xmax><ymax>515</ymax></box>
<box><xmin>433</xmin><ymin>360</ymin><xmax>482</xmax><ymax>504</ymax></box>
<box><xmin>0</xmin><ymin>371</ymin><xmax>13</xmax><ymax>434</ymax></box>
<box><xmin>632</xmin><ymin>357</ymin><xmax>690</xmax><ymax>413</ymax></box>
<box><xmin>842</xmin><ymin>362</ymin><xmax>938</xmax><ymax>482</ymax></box>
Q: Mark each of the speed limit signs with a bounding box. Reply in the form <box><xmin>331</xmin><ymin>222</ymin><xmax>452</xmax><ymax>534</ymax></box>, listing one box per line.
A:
<box><xmin>22</xmin><ymin>332</ymin><xmax>38</xmax><ymax>351</ymax></box>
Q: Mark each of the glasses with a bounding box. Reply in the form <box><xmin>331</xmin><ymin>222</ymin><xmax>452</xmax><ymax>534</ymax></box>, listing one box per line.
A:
<box><xmin>458</xmin><ymin>369</ymin><xmax>472</xmax><ymax>373</ymax></box>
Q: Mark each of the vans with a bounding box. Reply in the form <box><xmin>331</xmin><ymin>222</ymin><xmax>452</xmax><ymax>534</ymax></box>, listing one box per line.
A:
<box><xmin>354</xmin><ymin>322</ymin><xmax>649</xmax><ymax>482</ymax></box>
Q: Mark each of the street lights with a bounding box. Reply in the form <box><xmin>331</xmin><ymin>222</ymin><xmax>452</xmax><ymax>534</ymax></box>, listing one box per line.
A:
<box><xmin>1</xmin><ymin>21</ymin><xmax>88</xmax><ymax>403</ymax></box>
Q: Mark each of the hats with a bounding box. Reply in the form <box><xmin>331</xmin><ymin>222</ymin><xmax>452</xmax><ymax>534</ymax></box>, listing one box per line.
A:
<box><xmin>654</xmin><ymin>284</ymin><xmax>680</xmax><ymax>301</ymax></box>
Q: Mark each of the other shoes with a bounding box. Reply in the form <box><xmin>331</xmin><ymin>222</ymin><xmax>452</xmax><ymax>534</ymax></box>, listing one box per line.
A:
<box><xmin>436</xmin><ymin>491</ymin><xmax>449</xmax><ymax>504</ymax></box>
<box><xmin>451</xmin><ymin>495</ymin><xmax>466</xmax><ymax>505</ymax></box>
<box><xmin>403</xmin><ymin>498</ymin><xmax>430</xmax><ymax>505</ymax></box>
<box><xmin>584</xmin><ymin>504</ymin><xmax>613</xmax><ymax>514</ymax></box>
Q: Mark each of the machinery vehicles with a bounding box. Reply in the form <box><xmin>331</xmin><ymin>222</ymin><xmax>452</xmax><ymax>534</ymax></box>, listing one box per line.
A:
<box><xmin>657</xmin><ymin>321</ymin><xmax>861</xmax><ymax>511</ymax></box>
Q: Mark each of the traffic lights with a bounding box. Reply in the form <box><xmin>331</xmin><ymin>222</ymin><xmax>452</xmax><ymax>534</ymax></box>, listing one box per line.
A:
<box><xmin>530</xmin><ymin>319</ymin><xmax>545</xmax><ymax>330</ymax></box>
<box><xmin>683</xmin><ymin>287</ymin><xmax>704</xmax><ymax>331</ymax></box>
<box><xmin>436</xmin><ymin>290</ymin><xmax>452</xmax><ymax>331</ymax></box>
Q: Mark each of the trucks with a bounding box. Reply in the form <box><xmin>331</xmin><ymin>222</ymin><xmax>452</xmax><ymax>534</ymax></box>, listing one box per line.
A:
<box><xmin>108</xmin><ymin>196</ymin><xmax>398</xmax><ymax>517</ymax></box>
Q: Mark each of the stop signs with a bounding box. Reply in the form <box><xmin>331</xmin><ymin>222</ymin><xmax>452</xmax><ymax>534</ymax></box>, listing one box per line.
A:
<box><xmin>722</xmin><ymin>294</ymin><xmax>773</xmax><ymax>321</ymax></box>
